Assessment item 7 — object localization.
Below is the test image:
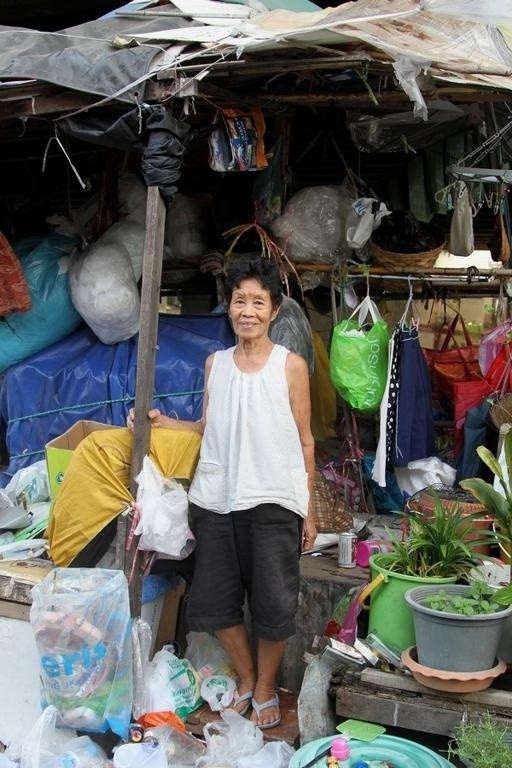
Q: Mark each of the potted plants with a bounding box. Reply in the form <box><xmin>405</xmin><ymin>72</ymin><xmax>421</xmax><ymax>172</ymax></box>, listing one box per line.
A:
<box><xmin>402</xmin><ymin>583</ymin><xmax>512</xmax><ymax>694</ymax></box>
<box><xmin>368</xmin><ymin>483</ymin><xmax>502</xmax><ymax>664</ymax></box>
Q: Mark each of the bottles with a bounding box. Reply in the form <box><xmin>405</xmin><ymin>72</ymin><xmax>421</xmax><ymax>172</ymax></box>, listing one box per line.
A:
<box><xmin>323</xmin><ymin>735</ymin><xmax>371</xmax><ymax>767</ymax></box>
<box><xmin>356</xmin><ymin>540</ymin><xmax>394</xmax><ymax>569</ymax></box>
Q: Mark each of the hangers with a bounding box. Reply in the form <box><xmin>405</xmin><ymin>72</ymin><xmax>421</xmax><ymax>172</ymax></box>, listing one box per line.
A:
<box><xmin>401</xmin><ymin>274</ymin><xmax>422</xmax><ymax>331</ymax></box>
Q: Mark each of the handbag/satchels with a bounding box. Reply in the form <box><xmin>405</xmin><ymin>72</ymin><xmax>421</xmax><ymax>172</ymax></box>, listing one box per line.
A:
<box><xmin>329</xmin><ymin>296</ymin><xmax>390</xmax><ymax>412</ymax></box>
<box><xmin>454</xmin><ymin>381</ymin><xmax>512</xmax><ymax>488</ymax></box>
<box><xmin>421</xmin><ymin>342</ymin><xmax>486</xmax><ymax>417</ymax></box>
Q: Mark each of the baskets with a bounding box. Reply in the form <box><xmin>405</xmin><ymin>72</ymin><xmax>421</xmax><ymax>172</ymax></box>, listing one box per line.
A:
<box><xmin>369</xmin><ymin>221</ymin><xmax>448</xmax><ymax>294</ymax></box>
<box><xmin>416</xmin><ymin>491</ymin><xmax>496</xmax><ymax>556</ymax></box>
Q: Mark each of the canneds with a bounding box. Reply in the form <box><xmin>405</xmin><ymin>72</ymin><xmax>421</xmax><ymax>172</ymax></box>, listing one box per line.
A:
<box><xmin>129</xmin><ymin>724</ymin><xmax>144</xmax><ymax>743</ymax></box>
<box><xmin>337</xmin><ymin>532</ymin><xmax>358</xmax><ymax>568</ymax></box>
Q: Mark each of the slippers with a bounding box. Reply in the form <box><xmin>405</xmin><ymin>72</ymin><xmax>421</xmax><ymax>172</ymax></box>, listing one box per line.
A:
<box><xmin>229</xmin><ymin>688</ymin><xmax>281</xmax><ymax>729</ymax></box>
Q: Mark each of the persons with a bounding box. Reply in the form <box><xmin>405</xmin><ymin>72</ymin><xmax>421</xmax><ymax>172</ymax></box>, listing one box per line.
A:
<box><xmin>122</xmin><ymin>252</ymin><xmax>321</xmax><ymax>731</ymax></box>
